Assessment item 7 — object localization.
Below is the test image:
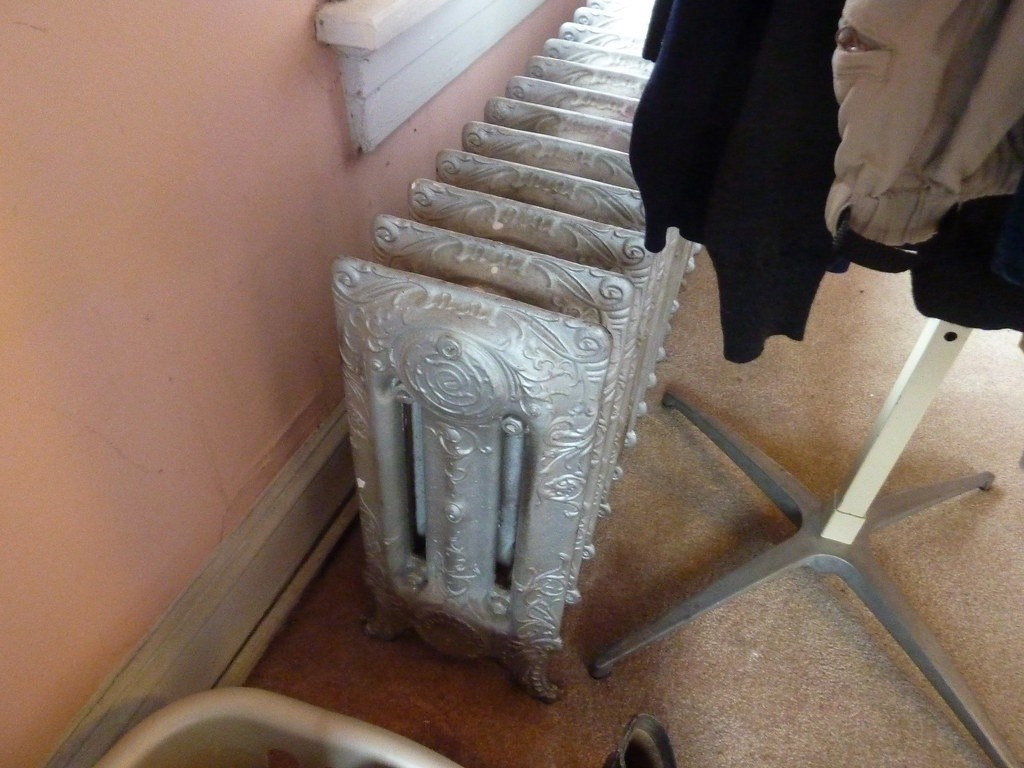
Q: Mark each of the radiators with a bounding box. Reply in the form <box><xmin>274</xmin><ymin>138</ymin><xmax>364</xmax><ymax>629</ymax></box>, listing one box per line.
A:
<box><xmin>327</xmin><ymin>0</ymin><xmax>706</xmax><ymax>698</ymax></box>
